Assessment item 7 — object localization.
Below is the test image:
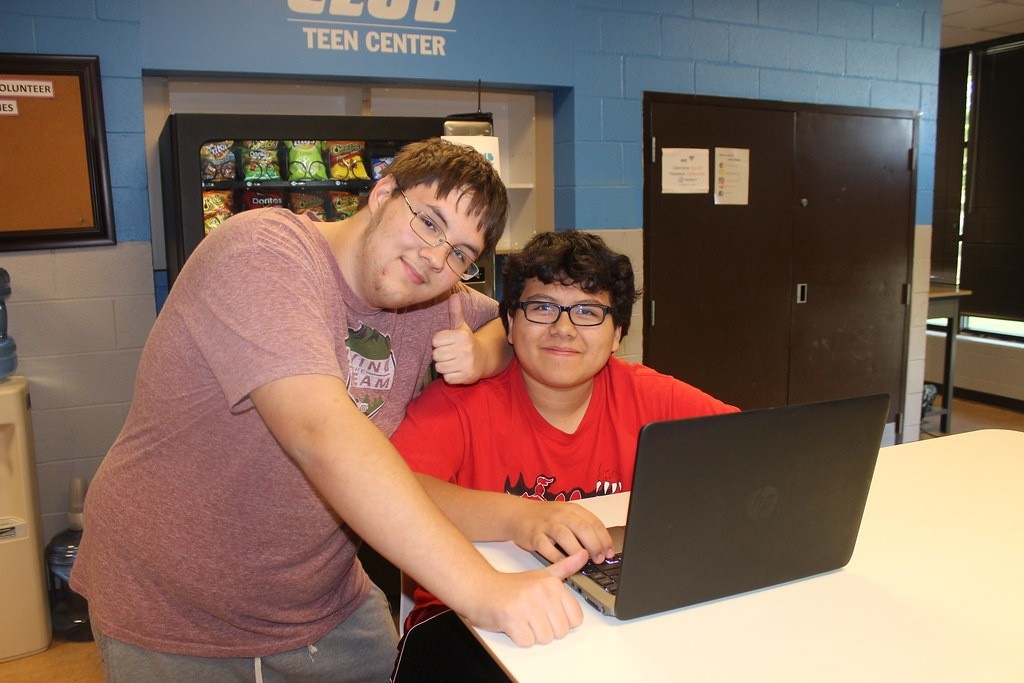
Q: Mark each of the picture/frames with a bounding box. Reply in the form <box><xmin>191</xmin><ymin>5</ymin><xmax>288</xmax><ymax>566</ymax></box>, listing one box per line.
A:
<box><xmin>0</xmin><ymin>52</ymin><xmax>120</xmax><ymax>252</ymax></box>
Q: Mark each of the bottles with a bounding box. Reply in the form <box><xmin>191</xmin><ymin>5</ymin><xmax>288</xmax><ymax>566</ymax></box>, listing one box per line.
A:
<box><xmin>43</xmin><ymin>478</ymin><xmax>94</xmax><ymax>641</ymax></box>
<box><xmin>0</xmin><ymin>267</ymin><xmax>17</xmax><ymax>379</ymax></box>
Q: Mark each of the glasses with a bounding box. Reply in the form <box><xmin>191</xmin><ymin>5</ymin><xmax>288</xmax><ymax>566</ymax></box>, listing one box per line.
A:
<box><xmin>515</xmin><ymin>301</ymin><xmax>613</xmax><ymax>326</ymax></box>
<box><xmin>397</xmin><ymin>183</ymin><xmax>479</xmax><ymax>280</ymax></box>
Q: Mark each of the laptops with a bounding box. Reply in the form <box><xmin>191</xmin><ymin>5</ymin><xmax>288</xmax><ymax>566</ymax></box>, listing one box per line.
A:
<box><xmin>531</xmin><ymin>393</ymin><xmax>892</xmax><ymax>622</ymax></box>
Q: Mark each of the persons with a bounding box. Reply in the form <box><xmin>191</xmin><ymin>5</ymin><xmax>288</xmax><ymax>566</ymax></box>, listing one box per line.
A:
<box><xmin>66</xmin><ymin>137</ymin><xmax>592</xmax><ymax>683</ymax></box>
<box><xmin>385</xmin><ymin>234</ymin><xmax>742</xmax><ymax>683</ymax></box>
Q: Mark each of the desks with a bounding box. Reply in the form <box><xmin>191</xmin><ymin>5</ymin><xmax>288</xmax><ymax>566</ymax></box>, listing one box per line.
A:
<box><xmin>921</xmin><ymin>286</ymin><xmax>973</xmax><ymax>437</ymax></box>
<box><xmin>458</xmin><ymin>429</ymin><xmax>1024</xmax><ymax>683</ymax></box>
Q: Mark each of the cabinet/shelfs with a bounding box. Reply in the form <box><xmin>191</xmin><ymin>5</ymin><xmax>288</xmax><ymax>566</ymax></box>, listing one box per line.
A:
<box><xmin>160</xmin><ymin>114</ymin><xmax>497</xmax><ymax>300</ymax></box>
